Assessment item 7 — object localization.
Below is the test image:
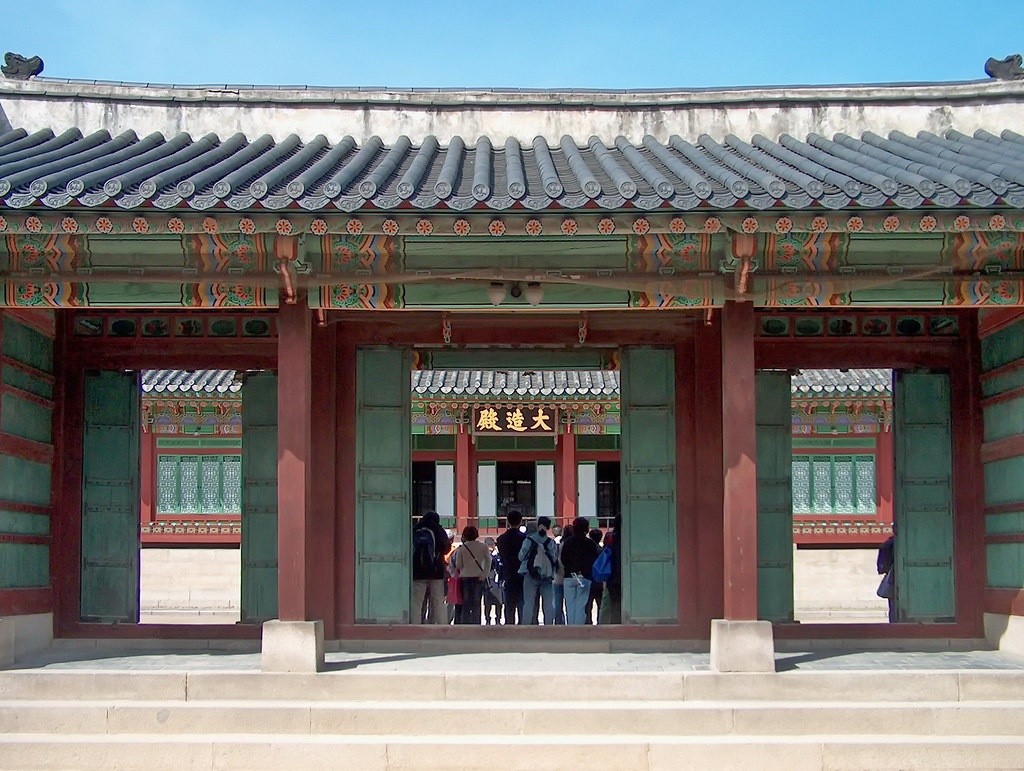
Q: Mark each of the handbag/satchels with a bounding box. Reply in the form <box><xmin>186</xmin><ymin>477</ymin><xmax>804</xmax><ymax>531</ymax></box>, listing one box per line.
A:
<box><xmin>877</xmin><ymin>562</ymin><xmax>894</xmax><ymax>598</ymax></box>
<box><xmin>569</xmin><ymin>573</ymin><xmax>584</xmax><ymax>587</ymax></box>
<box><xmin>591</xmin><ymin>545</ymin><xmax>612</xmax><ymax>583</ymax></box>
<box><xmin>448</xmin><ymin>567</ymin><xmax>464</xmax><ymax>604</ymax></box>
<box><xmin>482</xmin><ymin>577</ymin><xmax>492</xmax><ymax>592</ymax></box>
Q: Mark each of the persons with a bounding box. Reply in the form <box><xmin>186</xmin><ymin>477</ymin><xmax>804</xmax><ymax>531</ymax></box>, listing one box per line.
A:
<box><xmin>410</xmin><ymin>509</ymin><xmax>622</xmax><ymax>625</ymax></box>
<box><xmin>876</xmin><ymin>521</ymin><xmax>896</xmax><ymax>623</ymax></box>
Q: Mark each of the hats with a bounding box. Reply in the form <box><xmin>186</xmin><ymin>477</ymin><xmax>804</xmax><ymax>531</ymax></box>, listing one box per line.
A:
<box><xmin>445</xmin><ymin>529</ymin><xmax>455</xmax><ymax>538</ymax></box>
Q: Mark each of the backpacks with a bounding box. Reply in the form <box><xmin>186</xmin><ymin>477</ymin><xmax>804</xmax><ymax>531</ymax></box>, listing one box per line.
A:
<box><xmin>415</xmin><ymin>523</ymin><xmax>442</xmax><ymax>575</ymax></box>
<box><xmin>527</xmin><ymin>535</ymin><xmax>555</xmax><ymax>582</ymax></box>
